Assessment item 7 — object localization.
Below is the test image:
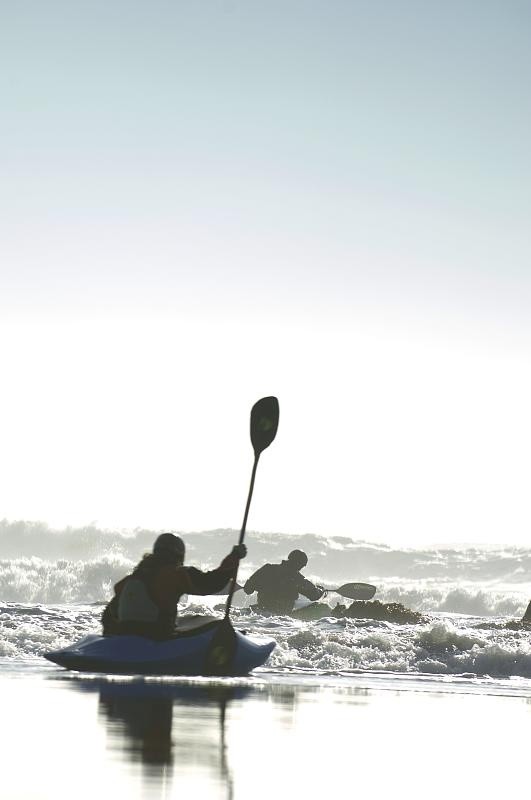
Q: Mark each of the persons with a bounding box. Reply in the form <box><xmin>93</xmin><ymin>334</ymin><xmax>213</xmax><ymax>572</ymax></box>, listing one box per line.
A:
<box><xmin>245</xmin><ymin>550</ymin><xmax>324</xmax><ymax>612</ymax></box>
<box><xmin>101</xmin><ymin>533</ymin><xmax>246</xmax><ymax>636</ymax></box>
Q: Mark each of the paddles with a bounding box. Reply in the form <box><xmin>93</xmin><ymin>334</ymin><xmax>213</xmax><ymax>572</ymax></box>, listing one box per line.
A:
<box><xmin>210</xmin><ymin>579</ymin><xmax>376</xmax><ymax>601</ymax></box>
<box><xmin>223</xmin><ymin>396</ymin><xmax>281</xmax><ymax>623</ymax></box>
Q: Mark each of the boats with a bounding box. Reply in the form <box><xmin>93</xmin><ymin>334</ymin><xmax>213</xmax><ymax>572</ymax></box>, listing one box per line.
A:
<box><xmin>43</xmin><ymin>612</ymin><xmax>277</xmax><ymax>678</ymax></box>
